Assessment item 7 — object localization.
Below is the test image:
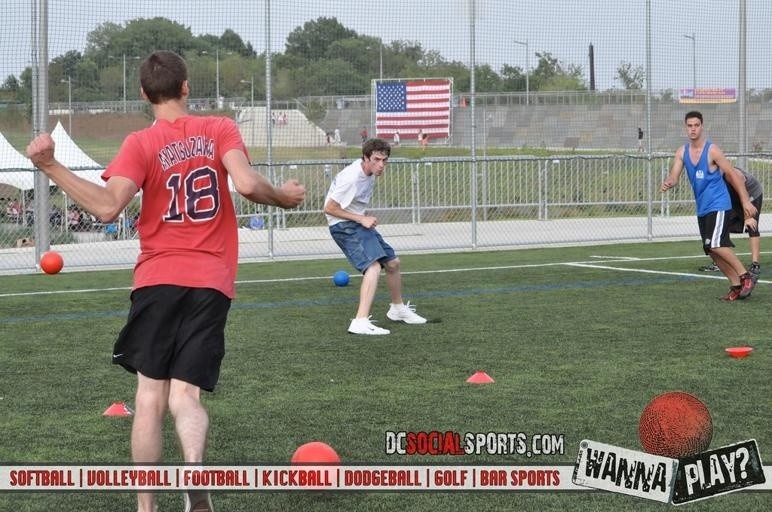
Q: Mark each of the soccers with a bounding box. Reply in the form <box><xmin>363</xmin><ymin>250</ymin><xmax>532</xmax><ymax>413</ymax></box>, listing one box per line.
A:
<box><xmin>41</xmin><ymin>254</ymin><xmax>64</xmax><ymax>272</ymax></box>
<box><xmin>639</xmin><ymin>391</ymin><xmax>714</xmax><ymax>456</ymax></box>
<box><xmin>334</xmin><ymin>270</ymin><xmax>350</xmax><ymax>285</ymax></box>
<box><xmin>292</xmin><ymin>442</ymin><xmax>341</xmax><ymax>466</ymax></box>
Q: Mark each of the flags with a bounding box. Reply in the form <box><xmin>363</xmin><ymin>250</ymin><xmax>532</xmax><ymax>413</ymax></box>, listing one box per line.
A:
<box><xmin>375</xmin><ymin>80</ymin><xmax>450</xmax><ymax>139</ymax></box>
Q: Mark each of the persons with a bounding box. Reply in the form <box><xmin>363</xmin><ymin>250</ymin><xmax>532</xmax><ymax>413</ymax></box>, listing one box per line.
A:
<box><xmin>637</xmin><ymin>127</ymin><xmax>647</xmax><ymax>153</ymax></box>
<box><xmin>696</xmin><ymin>157</ymin><xmax>764</xmax><ymax>281</ymax></box>
<box><xmin>0</xmin><ymin>195</ymin><xmax>141</xmax><ymax>247</ymax></box>
<box><xmin>658</xmin><ymin>109</ymin><xmax>761</xmax><ymax>302</ymax></box>
<box><xmin>276</xmin><ymin>111</ymin><xmax>284</xmax><ymax>127</ymax></box>
<box><xmin>422</xmin><ymin>133</ymin><xmax>430</xmax><ymax>150</ymax></box>
<box><xmin>271</xmin><ymin>110</ymin><xmax>276</xmax><ymax>126</ymax></box>
<box><xmin>325</xmin><ymin>131</ymin><xmax>331</xmax><ymax>146</ymax></box>
<box><xmin>334</xmin><ymin>126</ymin><xmax>342</xmax><ymax>142</ymax></box>
<box><xmin>186</xmin><ymin>92</ymin><xmax>225</xmax><ymax>112</ymax></box>
<box><xmin>284</xmin><ymin>112</ymin><xmax>289</xmax><ymax>126</ymax></box>
<box><xmin>321</xmin><ymin>138</ymin><xmax>429</xmax><ymax>337</ymax></box>
<box><xmin>360</xmin><ymin>124</ymin><xmax>368</xmax><ymax>144</ymax></box>
<box><xmin>392</xmin><ymin>128</ymin><xmax>402</xmax><ymax>147</ymax></box>
<box><xmin>22</xmin><ymin>45</ymin><xmax>309</xmax><ymax>512</ymax></box>
<box><xmin>417</xmin><ymin>128</ymin><xmax>423</xmax><ymax>148</ymax></box>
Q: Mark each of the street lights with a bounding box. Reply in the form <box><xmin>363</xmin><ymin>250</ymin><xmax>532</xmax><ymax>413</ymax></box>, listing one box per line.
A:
<box><xmin>681</xmin><ymin>32</ymin><xmax>696</xmax><ymax>89</ymax></box>
<box><xmin>60</xmin><ymin>77</ymin><xmax>72</xmax><ymax>136</ymax></box>
<box><xmin>240</xmin><ymin>77</ymin><xmax>254</xmax><ymax>106</ymax></box>
<box><xmin>513</xmin><ymin>38</ymin><xmax>529</xmax><ymax>104</ymax></box>
<box><xmin>108</xmin><ymin>54</ymin><xmax>141</xmax><ymax>112</ymax></box>
<box><xmin>202</xmin><ymin>49</ymin><xmax>232</xmax><ymax>98</ymax></box>
<box><xmin>366</xmin><ymin>37</ymin><xmax>382</xmax><ymax>84</ymax></box>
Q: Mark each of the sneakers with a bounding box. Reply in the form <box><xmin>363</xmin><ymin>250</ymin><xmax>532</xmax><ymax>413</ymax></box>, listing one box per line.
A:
<box><xmin>386</xmin><ymin>303</ymin><xmax>427</xmax><ymax>325</ymax></box>
<box><xmin>739</xmin><ymin>270</ymin><xmax>759</xmax><ymax>300</ymax></box>
<box><xmin>348</xmin><ymin>318</ymin><xmax>391</xmax><ymax>336</ymax></box>
<box><xmin>749</xmin><ymin>261</ymin><xmax>760</xmax><ymax>274</ymax></box>
<box><xmin>184</xmin><ymin>486</ymin><xmax>214</xmax><ymax>512</ymax></box>
<box><xmin>698</xmin><ymin>262</ymin><xmax>721</xmax><ymax>272</ymax></box>
<box><xmin>720</xmin><ymin>288</ymin><xmax>742</xmax><ymax>302</ymax></box>
